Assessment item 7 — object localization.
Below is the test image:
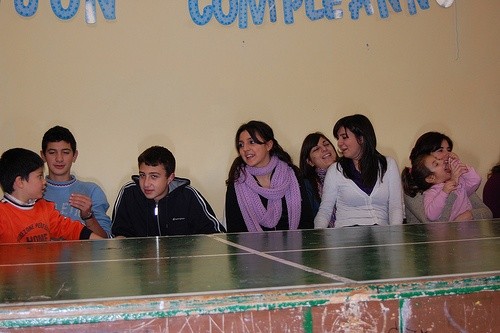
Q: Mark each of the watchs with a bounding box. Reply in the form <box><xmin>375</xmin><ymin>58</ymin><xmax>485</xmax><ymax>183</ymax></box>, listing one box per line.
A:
<box><xmin>81</xmin><ymin>211</ymin><xmax>95</xmax><ymax>220</ymax></box>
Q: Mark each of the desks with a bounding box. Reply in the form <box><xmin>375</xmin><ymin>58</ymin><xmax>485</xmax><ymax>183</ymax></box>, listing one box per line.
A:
<box><xmin>0</xmin><ymin>217</ymin><xmax>500</xmax><ymax>333</ymax></box>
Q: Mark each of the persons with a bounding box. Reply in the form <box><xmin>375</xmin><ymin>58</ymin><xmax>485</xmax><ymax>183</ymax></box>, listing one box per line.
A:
<box><xmin>0</xmin><ymin>125</ymin><xmax>112</xmax><ymax>244</ymax></box>
<box><xmin>222</xmin><ymin>114</ymin><xmax>404</xmax><ymax>233</ymax></box>
<box><xmin>109</xmin><ymin>145</ymin><xmax>227</xmax><ymax>239</ymax></box>
<box><xmin>401</xmin><ymin>132</ymin><xmax>500</xmax><ymax>224</ymax></box>
